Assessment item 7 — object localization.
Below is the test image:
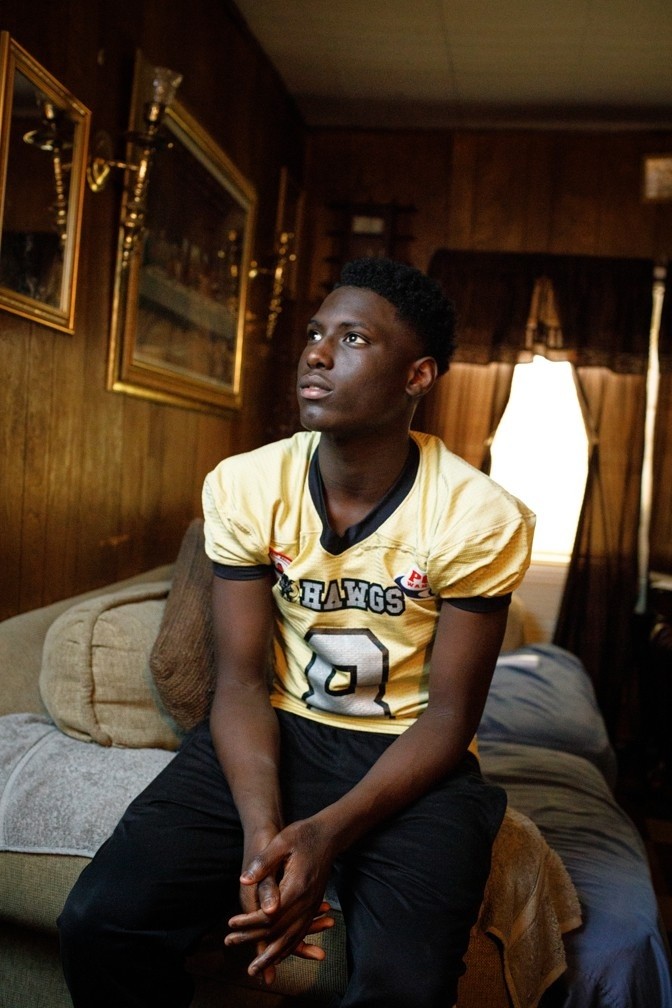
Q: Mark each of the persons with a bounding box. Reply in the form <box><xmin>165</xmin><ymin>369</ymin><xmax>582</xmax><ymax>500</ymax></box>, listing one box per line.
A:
<box><xmin>55</xmin><ymin>257</ymin><xmax>535</xmax><ymax>1008</ymax></box>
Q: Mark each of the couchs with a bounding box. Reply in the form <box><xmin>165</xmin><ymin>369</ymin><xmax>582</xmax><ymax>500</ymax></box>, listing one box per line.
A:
<box><xmin>0</xmin><ymin>523</ymin><xmax>672</xmax><ymax>1008</ymax></box>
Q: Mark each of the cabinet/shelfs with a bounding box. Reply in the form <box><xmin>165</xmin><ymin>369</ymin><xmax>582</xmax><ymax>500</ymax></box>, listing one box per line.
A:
<box><xmin>321</xmin><ymin>198</ymin><xmax>417</xmax><ymax>295</ymax></box>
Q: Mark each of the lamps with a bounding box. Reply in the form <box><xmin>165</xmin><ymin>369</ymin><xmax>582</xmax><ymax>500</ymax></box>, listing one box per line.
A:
<box><xmin>209</xmin><ymin>225</ymin><xmax>243</xmax><ymax>303</ymax></box>
<box><xmin>92</xmin><ymin>61</ymin><xmax>191</xmax><ymax>273</ymax></box>
<box><xmin>246</xmin><ymin>213</ymin><xmax>306</xmax><ymax>343</ymax></box>
<box><xmin>21</xmin><ymin>88</ymin><xmax>71</xmax><ymax>230</ymax></box>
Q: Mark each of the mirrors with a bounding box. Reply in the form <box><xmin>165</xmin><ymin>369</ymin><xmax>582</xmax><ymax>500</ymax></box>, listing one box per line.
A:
<box><xmin>100</xmin><ymin>44</ymin><xmax>260</xmax><ymax>421</ymax></box>
<box><xmin>0</xmin><ymin>27</ymin><xmax>95</xmax><ymax>336</ymax></box>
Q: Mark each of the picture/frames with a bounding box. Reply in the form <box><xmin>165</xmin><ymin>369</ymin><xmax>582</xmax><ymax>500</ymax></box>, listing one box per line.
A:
<box><xmin>275</xmin><ymin>164</ymin><xmax>308</xmax><ymax>258</ymax></box>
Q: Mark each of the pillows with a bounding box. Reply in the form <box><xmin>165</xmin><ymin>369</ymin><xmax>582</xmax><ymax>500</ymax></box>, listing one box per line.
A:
<box><xmin>152</xmin><ymin>511</ymin><xmax>272</xmax><ymax>722</ymax></box>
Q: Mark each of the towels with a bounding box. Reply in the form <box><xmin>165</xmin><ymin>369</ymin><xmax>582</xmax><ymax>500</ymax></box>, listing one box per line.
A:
<box><xmin>0</xmin><ymin>712</ymin><xmax>346</xmax><ymax>911</ymax></box>
<box><xmin>455</xmin><ymin>804</ymin><xmax>584</xmax><ymax>1008</ymax></box>
